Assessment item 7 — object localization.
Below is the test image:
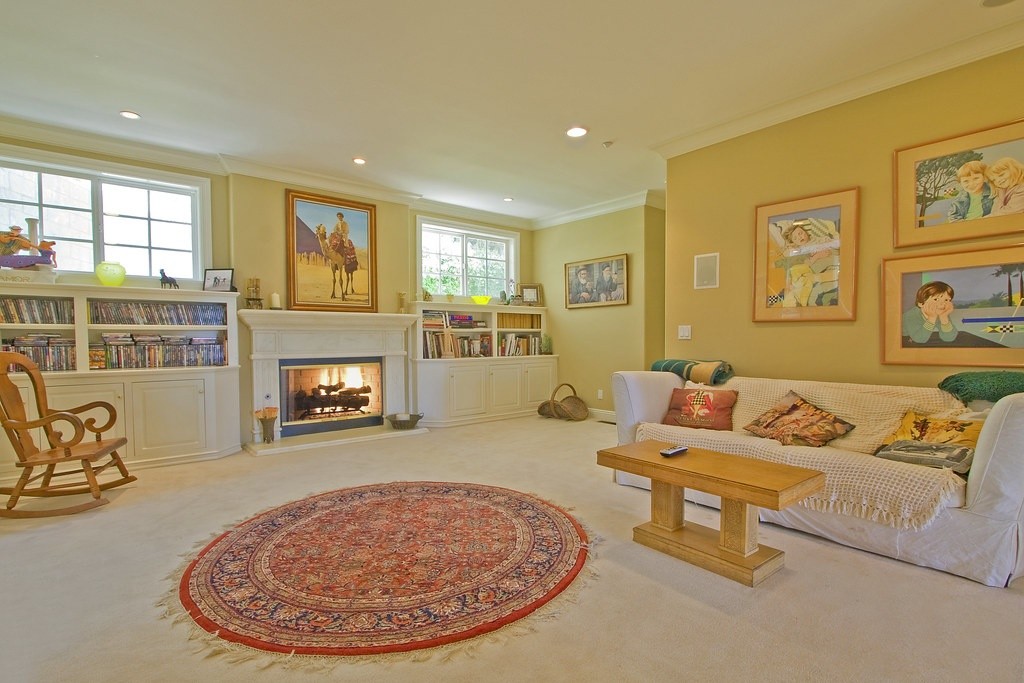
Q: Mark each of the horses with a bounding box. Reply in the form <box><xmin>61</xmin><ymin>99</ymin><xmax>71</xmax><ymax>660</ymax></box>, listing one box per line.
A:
<box><xmin>159</xmin><ymin>269</ymin><xmax>180</xmax><ymax>289</ymax></box>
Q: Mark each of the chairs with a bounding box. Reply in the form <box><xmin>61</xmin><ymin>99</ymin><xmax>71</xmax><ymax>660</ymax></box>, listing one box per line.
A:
<box><xmin>1</xmin><ymin>352</ymin><xmax>137</xmax><ymax>517</ymax></box>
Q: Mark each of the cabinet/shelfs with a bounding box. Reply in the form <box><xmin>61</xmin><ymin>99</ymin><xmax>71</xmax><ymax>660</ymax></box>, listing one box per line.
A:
<box><xmin>406</xmin><ymin>301</ymin><xmax>559</xmax><ymax>427</ymax></box>
<box><xmin>0</xmin><ymin>282</ymin><xmax>242</xmax><ymax>484</ymax></box>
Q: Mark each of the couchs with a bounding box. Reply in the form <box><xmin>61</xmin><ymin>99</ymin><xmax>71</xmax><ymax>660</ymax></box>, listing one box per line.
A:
<box><xmin>608</xmin><ymin>370</ymin><xmax>1024</xmax><ymax>587</ymax></box>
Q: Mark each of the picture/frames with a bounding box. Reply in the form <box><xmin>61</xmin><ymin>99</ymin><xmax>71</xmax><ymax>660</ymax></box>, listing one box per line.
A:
<box><xmin>564</xmin><ymin>253</ymin><xmax>628</xmax><ymax>309</ymax></box>
<box><xmin>202</xmin><ymin>268</ymin><xmax>234</xmax><ymax>291</ymax></box>
<box><xmin>283</xmin><ymin>187</ymin><xmax>378</xmax><ymax>312</ymax></box>
<box><xmin>891</xmin><ymin>117</ymin><xmax>1024</xmax><ymax>248</ymax></box>
<box><xmin>880</xmin><ymin>243</ymin><xmax>1024</xmax><ymax>368</ymax></box>
<box><xmin>518</xmin><ymin>283</ymin><xmax>542</xmax><ymax>306</ymax></box>
<box><xmin>752</xmin><ymin>186</ymin><xmax>860</xmax><ymax>322</ymax></box>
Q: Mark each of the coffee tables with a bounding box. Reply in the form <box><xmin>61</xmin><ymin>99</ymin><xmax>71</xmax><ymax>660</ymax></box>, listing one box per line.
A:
<box><xmin>597</xmin><ymin>438</ymin><xmax>826</xmax><ymax>588</ymax></box>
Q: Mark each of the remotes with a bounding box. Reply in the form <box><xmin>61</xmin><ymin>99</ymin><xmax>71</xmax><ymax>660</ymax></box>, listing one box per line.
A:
<box><xmin>660</xmin><ymin>446</ymin><xmax>688</xmax><ymax>458</ymax></box>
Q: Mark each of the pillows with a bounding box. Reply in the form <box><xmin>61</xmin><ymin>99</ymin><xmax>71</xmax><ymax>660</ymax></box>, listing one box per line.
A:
<box><xmin>883</xmin><ymin>407</ymin><xmax>985</xmax><ymax>448</ymax></box>
<box><xmin>937</xmin><ymin>371</ymin><xmax>1024</xmax><ymax>408</ymax></box>
<box><xmin>876</xmin><ymin>439</ymin><xmax>975</xmax><ymax>472</ymax></box>
<box><xmin>662</xmin><ymin>388</ymin><xmax>738</xmax><ymax>431</ymax></box>
<box><xmin>744</xmin><ymin>390</ymin><xmax>855</xmax><ymax>447</ymax></box>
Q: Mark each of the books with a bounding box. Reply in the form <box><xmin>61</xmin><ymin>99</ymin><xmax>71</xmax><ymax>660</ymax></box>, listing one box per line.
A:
<box><xmin>87</xmin><ymin>301</ymin><xmax>227</xmax><ymax>325</ymax></box>
<box><xmin>2</xmin><ymin>333</ymin><xmax>77</xmax><ymax>372</ymax></box>
<box><xmin>89</xmin><ymin>333</ymin><xmax>227</xmax><ymax>369</ymax></box>
<box><xmin>423</xmin><ymin>331</ymin><xmax>491</xmax><ymax>359</ymax></box>
<box><xmin>0</xmin><ymin>298</ymin><xmax>74</xmax><ymax>324</ymax></box>
<box><xmin>423</xmin><ymin>311</ymin><xmax>487</xmax><ymax>329</ymax></box>
<box><xmin>498</xmin><ymin>332</ymin><xmax>541</xmax><ymax>356</ymax></box>
<box><xmin>497</xmin><ymin>313</ymin><xmax>540</xmax><ymax>329</ymax></box>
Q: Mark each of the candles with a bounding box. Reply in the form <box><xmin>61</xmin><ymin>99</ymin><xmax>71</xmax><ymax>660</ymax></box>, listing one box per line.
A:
<box><xmin>396</xmin><ymin>413</ymin><xmax>410</xmax><ymax>420</ymax></box>
<box><xmin>272</xmin><ymin>292</ymin><xmax>281</xmax><ymax>307</ymax></box>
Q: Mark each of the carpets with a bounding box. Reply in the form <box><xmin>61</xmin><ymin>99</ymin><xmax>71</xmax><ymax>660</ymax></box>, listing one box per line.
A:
<box><xmin>156</xmin><ymin>481</ymin><xmax>596</xmax><ymax>674</ymax></box>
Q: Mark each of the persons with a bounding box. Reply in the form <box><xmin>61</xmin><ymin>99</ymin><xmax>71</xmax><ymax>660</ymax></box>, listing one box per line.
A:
<box><xmin>0</xmin><ymin>226</ymin><xmax>31</xmax><ymax>255</ymax></box>
